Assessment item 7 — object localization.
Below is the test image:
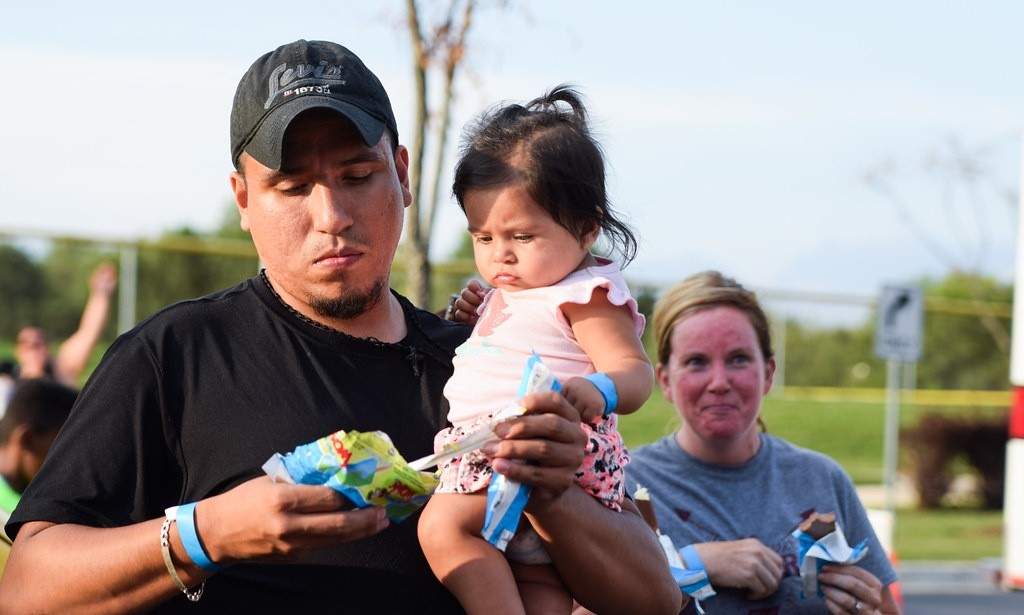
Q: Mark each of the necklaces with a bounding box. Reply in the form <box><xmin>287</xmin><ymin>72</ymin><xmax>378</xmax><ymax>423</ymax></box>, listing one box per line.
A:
<box><xmin>259</xmin><ymin>267</ymin><xmax>425</xmax><ymax>378</ymax></box>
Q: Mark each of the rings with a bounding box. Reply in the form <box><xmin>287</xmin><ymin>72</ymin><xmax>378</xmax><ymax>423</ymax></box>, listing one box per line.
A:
<box><xmin>845</xmin><ymin>597</ymin><xmax>864</xmax><ymax>615</ymax></box>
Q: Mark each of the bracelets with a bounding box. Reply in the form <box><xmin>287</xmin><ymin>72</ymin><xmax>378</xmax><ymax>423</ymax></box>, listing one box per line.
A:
<box><xmin>160</xmin><ymin>501</ymin><xmax>224</xmax><ymax>602</ymax></box>
<box><xmin>582</xmin><ymin>372</ymin><xmax>619</xmax><ymax>416</ymax></box>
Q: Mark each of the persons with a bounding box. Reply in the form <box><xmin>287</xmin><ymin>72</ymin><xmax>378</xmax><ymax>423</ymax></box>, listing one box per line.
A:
<box><xmin>416</xmin><ymin>83</ymin><xmax>655</xmax><ymax>615</ymax></box>
<box><xmin>0</xmin><ymin>376</ymin><xmax>82</xmax><ymax>581</ymax></box>
<box><xmin>0</xmin><ymin>260</ymin><xmax>119</xmax><ymax>422</ymax></box>
<box><xmin>0</xmin><ymin>39</ymin><xmax>683</xmax><ymax>615</ymax></box>
<box><xmin>624</xmin><ymin>270</ymin><xmax>904</xmax><ymax>615</ymax></box>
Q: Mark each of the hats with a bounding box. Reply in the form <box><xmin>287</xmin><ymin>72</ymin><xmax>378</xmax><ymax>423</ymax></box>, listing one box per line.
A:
<box><xmin>230</xmin><ymin>39</ymin><xmax>398</xmax><ymax>170</ymax></box>
